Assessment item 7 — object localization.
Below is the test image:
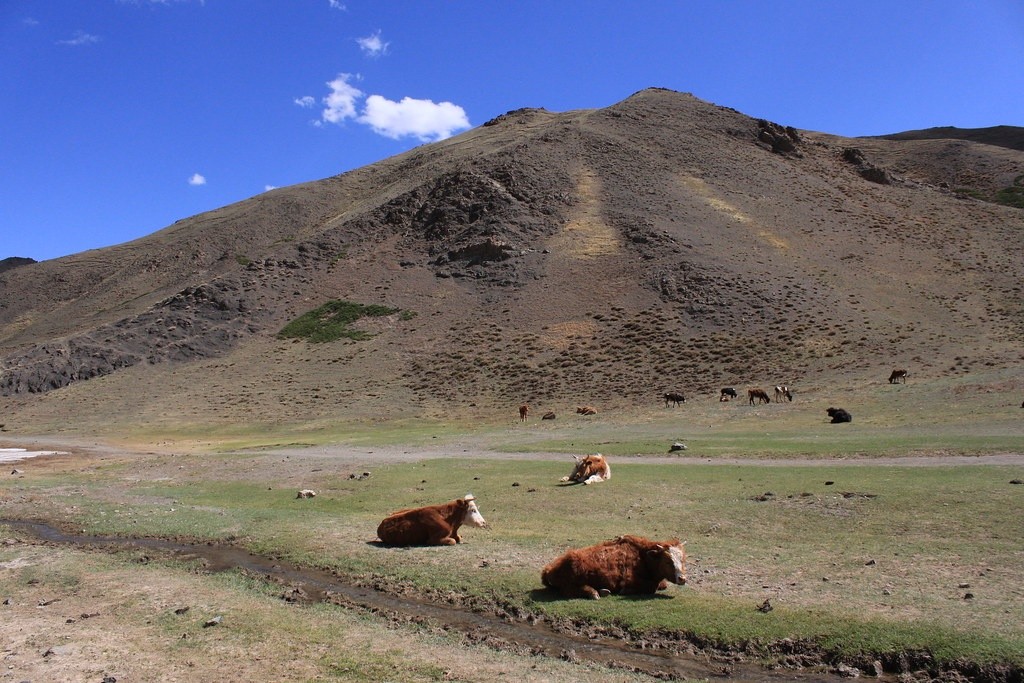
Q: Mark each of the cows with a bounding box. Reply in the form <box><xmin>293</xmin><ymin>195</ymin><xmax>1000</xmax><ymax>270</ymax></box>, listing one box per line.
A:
<box><xmin>519</xmin><ymin>405</ymin><xmax>529</xmax><ymax>422</ymax></box>
<box><xmin>376</xmin><ymin>494</ymin><xmax>487</xmax><ymax>547</ymax></box>
<box><xmin>888</xmin><ymin>369</ymin><xmax>908</xmax><ymax>384</ymax></box>
<box><xmin>569</xmin><ymin>452</ymin><xmax>611</xmax><ymax>485</ymax></box>
<box><xmin>719</xmin><ymin>386</ymin><xmax>793</xmax><ymax>405</ymax></box>
<box><xmin>663</xmin><ymin>393</ymin><xmax>686</xmax><ymax>408</ymax></box>
<box><xmin>577</xmin><ymin>406</ymin><xmax>598</xmax><ymax>416</ymax></box>
<box><xmin>541</xmin><ymin>533</ymin><xmax>688</xmax><ymax>601</ymax></box>
<box><xmin>825</xmin><ymin>407</ymin><xmax>852</xmax><ymax>424</ymax></box>
